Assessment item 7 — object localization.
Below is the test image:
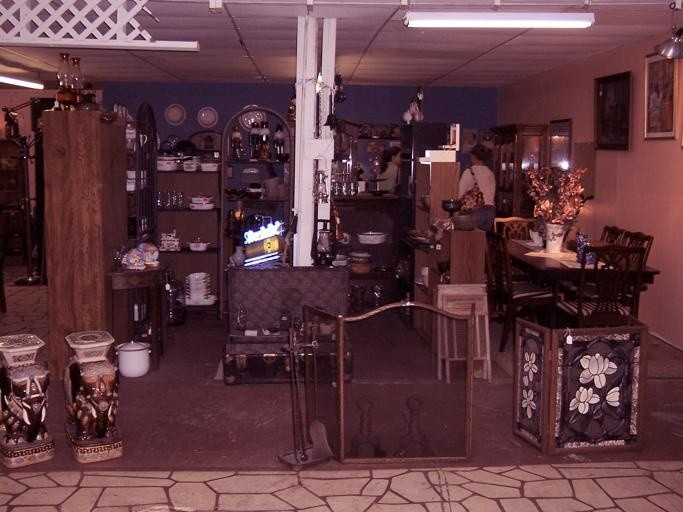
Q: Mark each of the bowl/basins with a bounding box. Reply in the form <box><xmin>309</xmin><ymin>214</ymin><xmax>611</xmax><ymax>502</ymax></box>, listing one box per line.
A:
<box><xmin>350</xmin><ymin>253</ymin><xmax>374</xmax><ymax>274</ymax></box>
<box><xmin>182</xmin><ymin>272</ymin><xmax>211</xmax><ymax>301</ymax></box>
<box><xmin>355</xmin><ymin>230</ymin><xmax>387</xmax><ymax>245</ymax></box>
<box><xmin>449</xmin><ymin>215</ymin><xmax>481</xmax><ymax>230</ymax></box>
<box><xmin>245</xmin><ymin>191</ymin><xmax>263</xmax><ymax>200</ymax></box>
<box><xmin>276</xmin><ymin>153</ymin><xmax>289</xmax><ymax>163</ymax></box>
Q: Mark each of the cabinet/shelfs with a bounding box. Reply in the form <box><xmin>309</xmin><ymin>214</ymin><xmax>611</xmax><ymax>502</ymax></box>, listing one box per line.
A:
<box><xmin>152</xmin><ymin>156</ymin><xmax>223</xmax><ymax>320</ymax></box>
<box><xmin>220</xmin><ymin>106</ymin><xmax>293</xmax><ymax>315</ymax></box>
<box><xmin>37</xmin><ymin>112</ymin><xmax>127</xmax><ymax>379</ymax></box>
<box><xmin>0</xmin><ymin>137</ymin><xmax>31</xmax><ymax>262</ymax></box>
<box><xmin>487</xmin><ymin>125</ymin><xmax>549</xmax><ymax>217</ymax></box>
<box><xmin>413</xmin><ymin>161</ymin><xmax>486</xmax><ymax>356</ymax></box>
<box><xmin>419</xmin><ymin>124</ymin><xmax>460</xmax><ymax>164</ymax></box>
<box><xmin>330</xmin><ymin>196</ymin><xmax>412</xmax><ymax>321</ymax></box>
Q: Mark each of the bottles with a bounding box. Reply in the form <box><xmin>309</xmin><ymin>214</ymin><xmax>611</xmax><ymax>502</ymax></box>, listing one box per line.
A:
<box><xmin>56</xmin><ymin>50</ymin><xmax>85</xmax><ymax>90</ymax></box>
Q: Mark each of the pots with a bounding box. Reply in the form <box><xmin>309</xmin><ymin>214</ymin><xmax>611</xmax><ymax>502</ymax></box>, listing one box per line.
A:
<box><xmin>126</xmin><ymin>123</ymin><xmax>146</xmax><ymax>153</ymax></box>
<box><xmin>188</xmin><ymin>193</ymin><xmax>214</xmax><ymax>209</ymax></box>
<box><xmin>157</xmin><ymin>152</ymin><xmax>219</xmax><ymax>172</ymax></box>
<box><xmin>184</xmin><ymin>241</ymin><xmax>211</xmax><ymax>252</ymax></box>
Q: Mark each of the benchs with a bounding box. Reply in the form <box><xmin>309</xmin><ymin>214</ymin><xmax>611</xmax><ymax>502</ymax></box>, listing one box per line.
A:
<box><xmin>220</xmin><ymin>265</ymin><xmax>351</xmax><ymax>385</ymax></box>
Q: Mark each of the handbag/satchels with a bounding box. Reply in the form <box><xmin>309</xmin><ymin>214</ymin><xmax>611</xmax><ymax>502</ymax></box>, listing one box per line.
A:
<box><xmin>456</xmin><ymin>167</ymin><xmax>485</xmax><ymax>216</ymax></box>
<box><xmin>395</xmin><ymin>166</ymin><xmax>401</xmax><ymax>196</ymax></box>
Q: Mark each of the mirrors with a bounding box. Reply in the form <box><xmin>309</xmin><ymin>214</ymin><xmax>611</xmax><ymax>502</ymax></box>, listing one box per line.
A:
<box><xmin>549</xmin><ymin>117</ymin><xmax>573</xmax><ymax>170</ymax></box>
<box><xmin>335</xmin><ymin>119</ymin><xmax>401</xmax><ymax>194</ymax></box>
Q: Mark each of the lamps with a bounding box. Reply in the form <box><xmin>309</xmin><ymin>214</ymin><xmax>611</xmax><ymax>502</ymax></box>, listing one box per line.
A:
<box><xmin>402</xmin><ymin>12</ymin><xmax>596</xmax><ymax>30</ymax></box>
<box><xmin>653</xmin><ymin>1</ymin><xmax>682</xmax><ymax>59</ymax></box>
<box><xmin>54</xmin><ymin>53</ymin><xmax>77</xmax><ymax>111</ymax></box>
<box><xmin>70</xmin><ymin>57</ymin><xmax>85</xmax><ymax>109</ymax></box>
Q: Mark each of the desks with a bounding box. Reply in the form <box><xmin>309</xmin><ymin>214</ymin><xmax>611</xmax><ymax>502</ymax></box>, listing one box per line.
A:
<box><xmin>111</xmin><ymin>259</ymin><xmax>170</xmax><ymax>361</ymax></box>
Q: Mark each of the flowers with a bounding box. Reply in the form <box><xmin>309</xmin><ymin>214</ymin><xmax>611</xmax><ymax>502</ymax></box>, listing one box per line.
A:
<box><xmin>398</xmin><ymin>85</ymin><xmax>425</xmax><ymax>124</ymax></box>
<box><xmin>525</xmin><ymin>166</ymin><xmax>592</xmax><ymax>233</ymax></box>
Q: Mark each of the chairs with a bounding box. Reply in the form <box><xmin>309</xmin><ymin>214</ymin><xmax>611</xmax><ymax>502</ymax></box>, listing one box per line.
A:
<box><xmin>556</xmin><ymin>224</ymin><xmax>623</xmax><ymax>293</ymax></box>
<box><xmin>483</xmin><ymin>232</ymin><xmax>531</xmax><ymax>303</ymax></box>
<box><xmin>493</xmin><ymin>216</ymin><xmax>537</xmax><ymax>240</ymax></box>
<box><xmin>484</xmin><ymin>231</ymin><xmax>562</xmax><ymax>352</ymax></box>
<box><xmin>554</xmin><ymin>241</ymin><xmax>645</xmax><ymax>328</ymax></box>
<box><xmin>576</xmin><ymin>230</ymin><xmax>652</xmax><ymax>319</ymax></box>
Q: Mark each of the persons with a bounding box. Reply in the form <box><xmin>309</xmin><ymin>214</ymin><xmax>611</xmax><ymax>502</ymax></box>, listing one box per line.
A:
<box><xmin>458</xmin><ymin>143</ymin><xmax>496</xmax><ymax>234</ymax></box>
<box><xmin>375</xmin><ymin>145</ymin><xmax>405</xmax><ymax>194</ymax></box>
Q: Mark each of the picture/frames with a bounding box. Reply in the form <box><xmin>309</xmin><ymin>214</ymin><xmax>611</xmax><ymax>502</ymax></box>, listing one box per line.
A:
<box><xmin>591</xmin><ymin>70</ymin><xmax>632</xmax><ymax>152</ymax></box>
<box><xmin>642</xmin><ymin>53</ymin><xmax>678</xmax><ymax>139</ymax></box>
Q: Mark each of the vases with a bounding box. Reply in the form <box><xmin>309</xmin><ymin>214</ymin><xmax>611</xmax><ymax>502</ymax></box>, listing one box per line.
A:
<box><xmin>402</xmin><ymin>104</ymin><xmax>423</xmax><ymax>123</ymax></box>
<box><xmin>62</xmin><ymin>330</ymin><xmax>125</xmax><ymax>463</ymax></box>
<box><xmin>0</xmin><ymin>334</ymin><xmax>55</xmax><ymax>468</ymax></box>
<box><xmin>545</xmin><ymin>217</ymin><xmax>570</xmax><ymax>253</ymax></box>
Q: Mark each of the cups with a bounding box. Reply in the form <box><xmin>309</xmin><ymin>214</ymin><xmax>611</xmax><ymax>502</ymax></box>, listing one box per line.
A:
<box><xmin>154</xmin><ymin>190</ymin><xmax>184</xmax><ymax>209</ymax></box>
<box><xmin>333</xmin><ymin>181</ymin><xmax>359</xmax><ymax>196</ymax></box>
